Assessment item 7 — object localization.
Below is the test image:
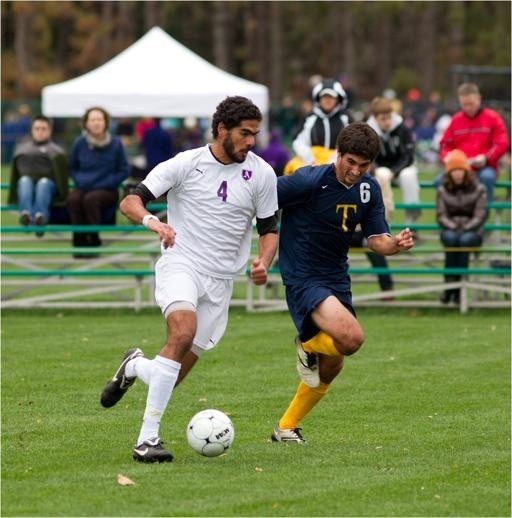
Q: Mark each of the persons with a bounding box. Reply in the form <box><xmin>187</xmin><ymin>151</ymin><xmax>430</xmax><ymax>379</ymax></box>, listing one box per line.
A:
<box><xmin>437</xmin><ymin>151</ymin><xmax>487</xmax><ymax>304</ymax></box>
<box><xmin>2</xmin><ymin>103</ymin><xmax>213</xmax><ymax>259</ymax></box>
<box><xmin>249</xmin><ymin>73</ymin><xmax>510</xmax><ymax>261</ymax></box>
<box><xmin>98</xmin><ymin>94</ymin><xmax>279</xmax><ymax>460</ymax></box>
<box><xmin>271</xmin><ymin>122</ymin><xmax>415</xmax><ymax>442</ymax></box>
<box><xmin>366</xmin><ymin>251</ymin><xmax>394</xmax><ymax>303</ymax></box>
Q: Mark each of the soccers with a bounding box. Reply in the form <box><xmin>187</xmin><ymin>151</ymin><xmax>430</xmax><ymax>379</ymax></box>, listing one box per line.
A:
<box><xmin>187</xmin><ymin>409</ymin><xmax>235</xmax><ymax>456</ymax></box>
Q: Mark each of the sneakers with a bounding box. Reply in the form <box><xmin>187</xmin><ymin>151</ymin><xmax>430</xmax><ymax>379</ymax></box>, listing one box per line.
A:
<box><xmin>271</xmin><ymin>422</ymin><xmax>307</xmax><ymax>447</ymax></box>
<box><xmin>294</xmin><ymin>334</ymin><xmax>320</xmax><ymax>389</ymax></box>
<box><xmin>102</xmin><ymin>347</ymin><xmax>145</xmax><ymax>406</ymax></box>
<box><xmin>133</xmin><ymin>438</ymin><xmax>172</xmax><ymax>462</ymax></box>
<box><xmin>17</xmin><ymin>209</ymin><xmax>44</xmax><ymax>238</ymax></box>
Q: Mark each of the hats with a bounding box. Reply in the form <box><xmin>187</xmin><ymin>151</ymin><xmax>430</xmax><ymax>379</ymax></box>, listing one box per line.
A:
<box><xmin>446</xmin><ymin>149</ymin><xmax>470</xmax><ymax>178</ymax></box>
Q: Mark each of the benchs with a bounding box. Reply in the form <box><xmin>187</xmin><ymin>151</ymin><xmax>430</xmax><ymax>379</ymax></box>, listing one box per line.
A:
<box><xmin>1</xmin><ymin>169</ymin><xmax>510</xmax><ymax>316</ymax></box>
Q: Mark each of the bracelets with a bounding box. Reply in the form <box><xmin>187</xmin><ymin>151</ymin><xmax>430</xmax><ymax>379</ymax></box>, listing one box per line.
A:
<box><xmin>142</xmin><ymin>214</ymin><xmax>158</xmax><ymax>228</ymax></box>
